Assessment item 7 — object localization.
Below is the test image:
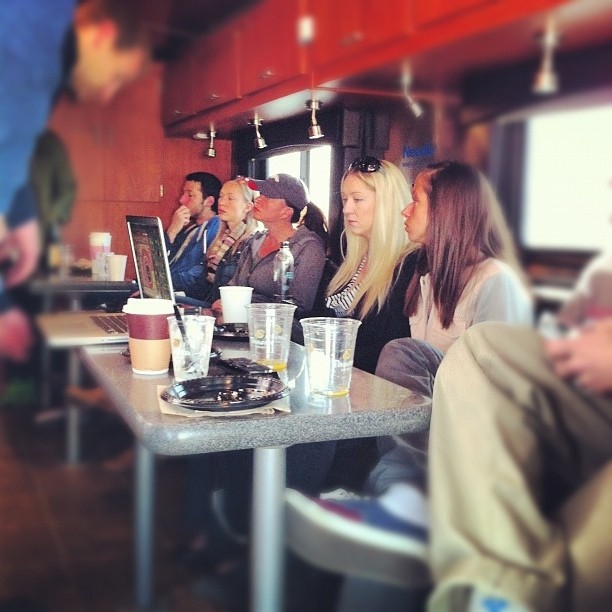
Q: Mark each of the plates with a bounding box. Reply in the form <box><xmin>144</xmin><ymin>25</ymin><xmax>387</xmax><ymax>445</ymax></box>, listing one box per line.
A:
<box><xmin>213</xmin><ymin>323</ymin><xmax>248</xmax><ymax>340</ymax></box>
<box><xmin>160</xmin><ymin>376</ymin><xmax>287</xmax><ymax>411</ymax></box>
<box><xmin>121</xmin><ymin>345</ymin><xmax>224</xmax><ymax>362</ymax></box>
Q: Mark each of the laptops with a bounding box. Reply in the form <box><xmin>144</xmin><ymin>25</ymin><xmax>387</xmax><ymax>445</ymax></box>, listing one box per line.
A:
<box><xmin>36</xmin><ymin>215</ymin><xmax>176</xmax><ymax>349</ymax></box>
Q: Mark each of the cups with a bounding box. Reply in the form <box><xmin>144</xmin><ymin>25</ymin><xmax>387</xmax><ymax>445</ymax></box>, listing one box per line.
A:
<box><xmin>166</xmin><ymin>315</ymin><xmax>216</xmax><ymax>383</ymax></box>
<box><xmin>108</xmin><ymin>252</ymin><xmax>128</xmax><ymax>282</ymax></box>
<box><xmin>297</xmin><ymin>317</ymin><xmax>363</xmax><ymax>397</ymax></box>
<box><xmin>248</xmin><ymin>303</ymin><xmax>298</xmax><ymax>371</ymax></box>
<box><xmin>121</xmin><ymin>298</ymin><xmax>177</xmax><ymax>375</ymax></box>
<box><xmin>218</xmin><ymin>286</ymin><xmax>255</xmax><ymax>324</ymax></box>
<box><xmin>89</xmin><ymin>231</ymin><xmax>110</xmax><ymax>281</ymax></box>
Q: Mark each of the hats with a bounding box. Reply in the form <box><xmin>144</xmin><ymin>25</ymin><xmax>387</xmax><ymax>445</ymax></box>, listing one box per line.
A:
<box><xmin>248</xmin><ymin>173</ymin><xmax>311</xmax><ymax>210</ymax></box>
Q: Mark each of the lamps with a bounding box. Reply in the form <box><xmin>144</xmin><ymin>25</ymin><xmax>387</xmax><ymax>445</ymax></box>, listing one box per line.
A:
<box><xmin>250</xmin><ymin>119</ymin><xmax>269</xmax><ymax>150</ymax></box>
<box><xmin>401</xmin><ymin>82</ymin><xmax>424</xmax><ymax>124</ymax></box>
<box><xmin>533</xmin><ymin>35</ymin><xmax>563</xmax><ymax>96</ymax></box>
<box><xmin>307</xmin><ymin>99</ymin><xmax>325</xmax><ymax>141</ymax></box>
<box><xmin>203</xmin><ymin>131</ymin><xmax>218</xmax><ymax>158</ymax></box>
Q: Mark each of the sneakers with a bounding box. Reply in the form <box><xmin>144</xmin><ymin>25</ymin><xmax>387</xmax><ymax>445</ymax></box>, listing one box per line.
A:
<box><xmin>286</xmin><ymin>489</ymin><xmax>431</xmax><ymax>592</ymax></box>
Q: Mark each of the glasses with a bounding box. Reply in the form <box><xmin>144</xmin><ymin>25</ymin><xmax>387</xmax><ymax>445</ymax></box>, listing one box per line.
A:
<box><xmin>348</xmin><ymin>155</ymin><xmax>388</xmax><ymax>178</ymax></box>
<box><xmin>235</xmin><ymin>174</ymin><xmax>254</xmax><ymax>203</ymax></box>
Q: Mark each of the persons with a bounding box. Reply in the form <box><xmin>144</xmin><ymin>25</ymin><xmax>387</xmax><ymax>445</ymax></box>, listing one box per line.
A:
<box><xmin>404</xmin><ymin>158</ymin><xmax>532</xmax><ymax>360</ymax></box>
<box><xmin>199</xmin><ymin>175</ymin><xmax>263</xmax><ymax>315</ymax></box>
<box><xmin>426</xmin><ymin>317</ymin><xmax>612</xmax><ymax>612</ymax></box>
<box><xmin>64</xmin><ymin>173</ymin><xmax>328</xmax><ymax>417</ymax></box>
<box><xmin>164</xmin><ymin>172</ymin><xmax>221</xmax><ymax>295</ymax></box>
<box><xmin>312</xmin><ymin>154</ymin><xmax>422</xmax><ymax>374</ymax></box>
<box><xmin>0</xmin><ymin>0</ymin><xmax>151</xmax><ymax>364</ymax></box>
<box><xmin>284</xmin><ymin>260</ymin><xmax>612</xmax><ymax>609</ymax></box>
